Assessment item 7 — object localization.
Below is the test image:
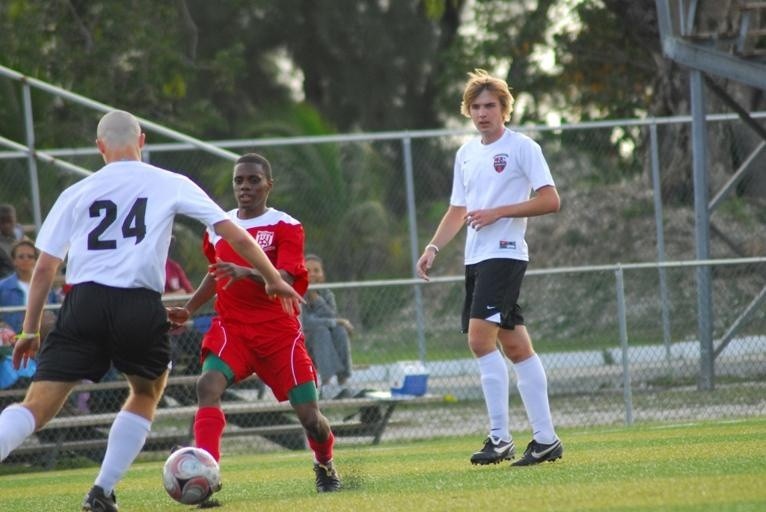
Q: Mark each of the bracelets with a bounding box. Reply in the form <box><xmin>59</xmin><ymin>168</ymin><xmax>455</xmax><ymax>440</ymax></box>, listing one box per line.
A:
<box><xmin>425</xmin><ymin>243</ymin><xmax>440</xmax><ymax>258</ymax></box>
<box><xmin>19</xmin><ymin>331</ymin><xmax>40</xmax><ymax>339</ymax></box>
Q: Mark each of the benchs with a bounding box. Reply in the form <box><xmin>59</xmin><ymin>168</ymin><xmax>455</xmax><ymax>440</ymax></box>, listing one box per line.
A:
<box><xmin>0</xmin><ymin>371</ymin><xmax>447</xmax><ymax>464</ymax></box>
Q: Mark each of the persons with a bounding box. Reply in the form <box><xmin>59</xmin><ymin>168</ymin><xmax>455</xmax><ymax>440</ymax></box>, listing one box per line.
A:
<box><xmin>0</xmin><ymin>203</ymin><xmax>308</xmax><ymax>449</ymax></box>
<box><xmin>298</xmin><ymin>255</ymin><xmax>364</xmax><ymax>398</ymax></box>
<box><xmin>159</xmin><ymin>152</ymin><xmax>344</xmax><ymax>495</ymax></box>
<box><xmin>412</xmin><ymin>77</ymin><xmax>565</xmax><ymax>466</ymax></box>
<box><xmin>0</xmin><ymin>108</ymin><xmax>308</xmax><ymax>512</ymax></box>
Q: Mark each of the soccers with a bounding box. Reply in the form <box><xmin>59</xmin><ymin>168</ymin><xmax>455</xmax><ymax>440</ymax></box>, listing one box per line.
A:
<box><xmin>162</xmin><ymin>447</ymin><xmax>219</xmax><ymax>504</ymax></box>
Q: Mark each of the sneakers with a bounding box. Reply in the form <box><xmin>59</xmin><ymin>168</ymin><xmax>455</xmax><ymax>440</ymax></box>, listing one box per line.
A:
<box><xmin>513</xmin><ymin>435</ymin><xmax>563</xmax><ymax>465</ymax></box>
<box><xmin>471</xmin><ymin>433</ymin><xmax>515</xmax><ymax>465</ymax></box>
<box><xmin>313</xmin><ymin>459</ymin><xmax>340</xmax><ymax>492</ymax></box>
<box><xmin>82</xmin><ymin>486</ymin><xmax>120</xmax><ymax>512</ymax></box>
